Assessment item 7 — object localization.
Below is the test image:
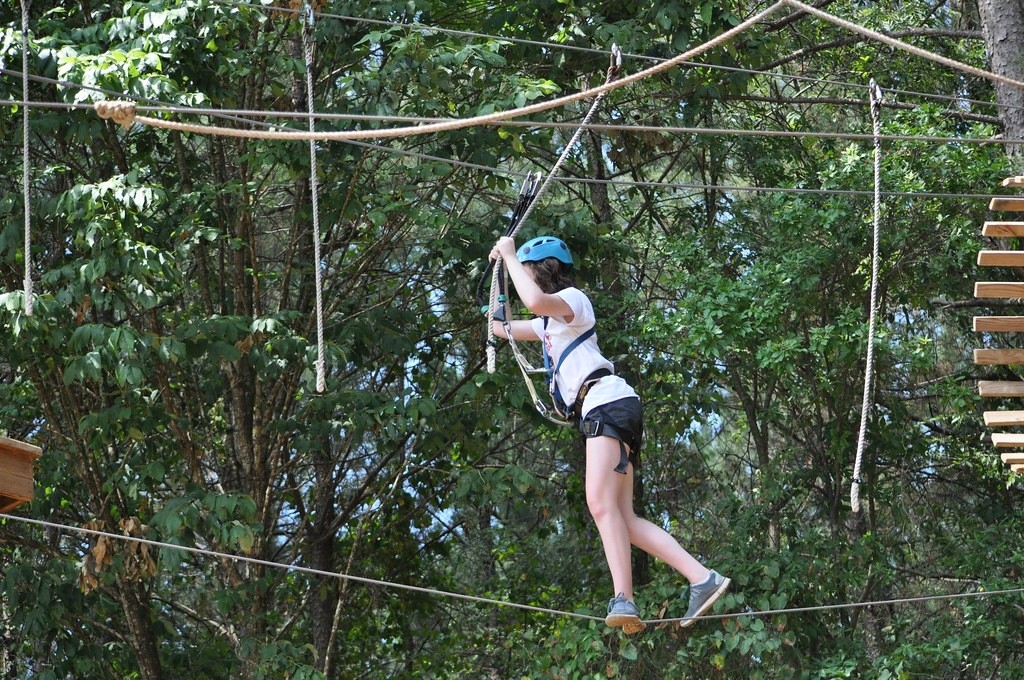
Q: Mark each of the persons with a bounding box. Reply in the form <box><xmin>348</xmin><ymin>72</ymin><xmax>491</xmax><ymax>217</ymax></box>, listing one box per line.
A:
<box><xmin>489</xmin><ymin>238</ymin><xmax>731</xmax><ymax>634</ymax></box>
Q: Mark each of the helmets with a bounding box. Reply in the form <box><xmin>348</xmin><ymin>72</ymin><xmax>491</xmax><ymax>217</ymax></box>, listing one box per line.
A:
<box><xmin>516</xmin><ymin>236</ymin><xmax>575</xmax><ymax>267</ymax></box>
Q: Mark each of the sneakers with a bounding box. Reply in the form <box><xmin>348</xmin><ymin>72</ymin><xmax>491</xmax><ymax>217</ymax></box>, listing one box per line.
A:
<box><xmin>605</xmin><ymin>592</ymin><xmax>646</xmax><ymax>634</ymax></box>
<box><xmin>680</xmin><ymin>569</ymin><xmax>730</xmax><ymax>627</ymax></box>
